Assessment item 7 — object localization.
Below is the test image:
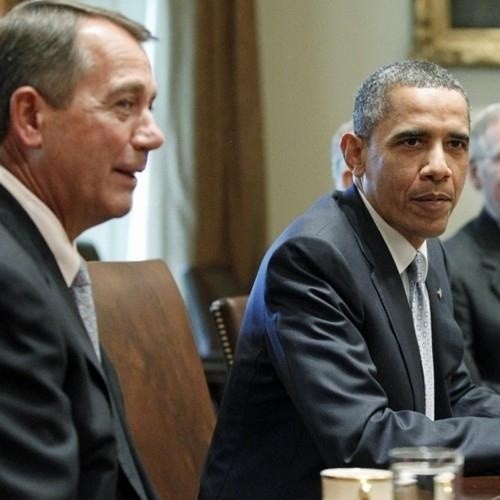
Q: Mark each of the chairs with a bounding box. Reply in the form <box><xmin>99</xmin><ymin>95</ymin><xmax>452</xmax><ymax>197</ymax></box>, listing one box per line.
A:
<box><xmin>85</xmin><ymin>255</ymin><xmax>224</xmax><ymax>500</ymax></box>
<box><xmin>208</xmin><ymin>290</ymin><xmax>247</xmax><ymax>364</ymax></box>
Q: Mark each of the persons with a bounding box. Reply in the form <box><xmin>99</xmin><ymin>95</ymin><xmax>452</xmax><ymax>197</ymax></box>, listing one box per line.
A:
<box><xmin>441</xmin><ymin>104</ymin><xmax>500</xmax><ymax>397</ymax></box>
<box><xmin>0</xmin><ymin>0</ymin><xmax>163</xmax><ymax>500</ymax></box>
<box><xmin>198</xmin><ymin>58</ymin><xmax>500</xmax><ymax>500</ymax></box>
<box><xmin>331</xmin><ymin>121</ymin><xmax>359</xmax><ymax>193</ymax></box>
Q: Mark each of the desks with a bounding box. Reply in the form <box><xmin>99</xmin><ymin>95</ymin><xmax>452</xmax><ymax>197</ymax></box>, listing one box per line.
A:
<box><xmin>388</xmin><ymin>456</ymin><xmax>499</xmax><ymax>499</ymax></box>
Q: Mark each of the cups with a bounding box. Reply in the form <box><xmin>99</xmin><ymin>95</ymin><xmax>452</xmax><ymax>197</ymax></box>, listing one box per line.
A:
<box><xmin>320</xmin><ymin>468</ymin><xmax>394</xmax><ymax>500</ymax></box>
<box><xmin>388</xmin><ymin>446</ymin><xmax>465</xmax><ymax>500</ymax></box>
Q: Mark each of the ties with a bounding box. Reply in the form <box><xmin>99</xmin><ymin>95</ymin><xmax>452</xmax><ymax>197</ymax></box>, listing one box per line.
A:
<box><xmin>405</xmin><ymin>251</ymin><xmax>436</xmax><ymax>423</ymax></box>
<box><xmin>71</xmin><ymin>263</ymin><xmax>102</xmax><ymax>364</ymax></box>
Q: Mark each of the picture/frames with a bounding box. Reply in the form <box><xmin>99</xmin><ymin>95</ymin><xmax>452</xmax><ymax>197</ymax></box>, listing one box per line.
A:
<box><xmin>410</xmin><ymin>0</ymin><xmax>500</xmax><ymax>71</ymax></box>
